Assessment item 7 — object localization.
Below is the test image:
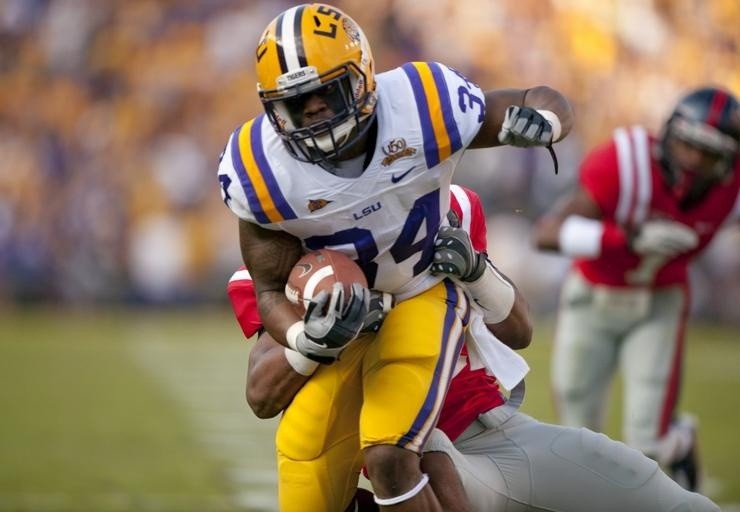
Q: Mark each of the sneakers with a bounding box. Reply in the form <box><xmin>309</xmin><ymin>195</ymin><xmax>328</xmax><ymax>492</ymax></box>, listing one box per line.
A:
<box><xmin>665</xmin><ymin>414</ymin><xmax>699</xmax><ymax>491</ymax></box>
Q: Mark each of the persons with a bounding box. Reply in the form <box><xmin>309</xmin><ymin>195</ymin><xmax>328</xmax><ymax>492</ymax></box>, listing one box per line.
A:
<box><xmin>526</xmin><ymin>87</ymin><xmax>740</xmax><ymax>493</ymax></box>
<box><xmin>226</xmin><ymin>183</ymin><xmax>724</xmax><ymax>512</ymax></box>
<box><xmin>217</xmin><ymin>3</ymin><xmax>573</xmax><ymax>512</ymax></box>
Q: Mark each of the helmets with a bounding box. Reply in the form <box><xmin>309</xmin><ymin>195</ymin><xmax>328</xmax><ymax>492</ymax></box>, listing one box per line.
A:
<box><xmin>663</xmin><ymin>89</ymin><xmax>740</xmax><ymax>186</ymax></box>
<box><xmin>256</xmin><ymin>4</ymin><xmax>378</xmax><ymax>163</ymax></box>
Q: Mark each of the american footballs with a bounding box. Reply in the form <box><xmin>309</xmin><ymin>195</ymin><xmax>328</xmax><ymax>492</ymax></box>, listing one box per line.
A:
<box><xmin>283</xmin><ymin>248</ymin><xmax>369</xmax><ymax>321</ymax></box>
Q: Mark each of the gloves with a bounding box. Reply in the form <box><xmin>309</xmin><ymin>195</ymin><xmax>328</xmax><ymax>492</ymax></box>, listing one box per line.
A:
<box><xmin>295</xmin><ymin>283</ymin><xmax>392</xmax><ymax>365</ymax></box>
<box><xmin>430</xmin><ymin>209</ymin><xmax>487</xmax><ymax>282</ymax></box>
<box><xmin>498</xmin><ymin>105</ymin><xmax>553</xmax><ymax>150</ymax></box>
<box><xmin>632</xmin><ymin>219</ymin><xmax>698</xmax><ymax>259</ymax></box>
